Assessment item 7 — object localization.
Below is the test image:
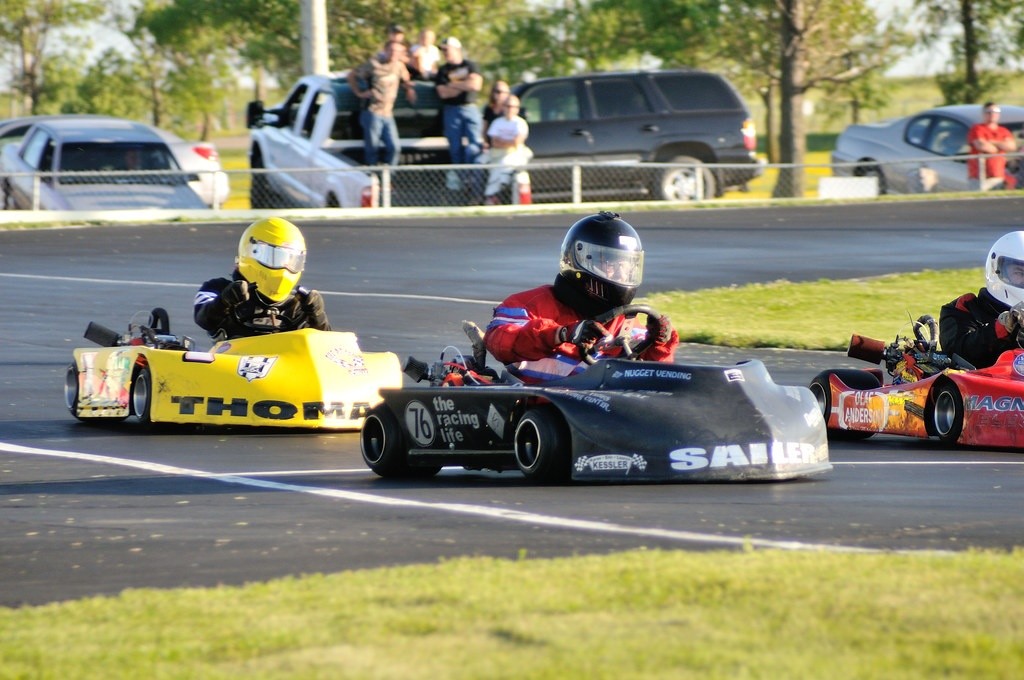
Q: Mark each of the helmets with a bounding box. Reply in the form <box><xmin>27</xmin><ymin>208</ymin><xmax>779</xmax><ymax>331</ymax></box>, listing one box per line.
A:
<box><xmin>986</xmin><ymin>231</ymin><xmax>1024</xmax><ymax>311</ymax></box>
<box><xmin>560</xmin><ymin>211</ymin><xmax>644</xmax><ymax>305</ymax></box>
<box><xmin>234</xmin><ymin>217</ymin><xmax>306</xmax><ymax>301</ymax></box>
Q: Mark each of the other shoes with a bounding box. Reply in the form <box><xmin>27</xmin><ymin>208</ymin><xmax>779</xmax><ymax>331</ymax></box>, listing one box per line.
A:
<box><xmin>445</xmin><ymin>169</ymin><xmax>461</xmax><ymax>190</ymax></box>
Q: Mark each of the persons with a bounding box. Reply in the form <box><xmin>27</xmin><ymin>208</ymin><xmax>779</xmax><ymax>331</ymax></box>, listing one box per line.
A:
<box><xmin>486</xmin><ymin>212</ymin><xmax>680</xmax><ymax>377</ymax></box>
<box><xmin>193</xmin><ymin>216</ymin><xmax>330</xmax><ymax>344</ymax></box>
<box><xmin>949</xmin><ymin>229</ymin><xmax>1024</xmax><ymax>365</ymax></box>
<box><xmin>966</xmin><ymin>100</ymin><xmax>1018</xmax><ymax>189</ymax></box>
<box><xmin>351</xmin><ymin>25</ymin><xmax>532</xmax><ymax>206</ymax></box>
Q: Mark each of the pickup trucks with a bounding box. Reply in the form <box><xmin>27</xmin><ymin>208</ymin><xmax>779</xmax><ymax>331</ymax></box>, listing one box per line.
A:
<box><xmin>247</xmin><ymin>68</ymin><xmax>534</xmax><ymax>208</ymax></box>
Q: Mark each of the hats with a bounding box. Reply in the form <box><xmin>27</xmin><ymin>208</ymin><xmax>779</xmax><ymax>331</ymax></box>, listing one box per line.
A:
<box><xmin>437</xmin><ymin>37</ymin><xmax>461</xmax><ymax>49</ymax></box>
<box><xmin>384</xmin><ymin>22</ymin><xmax>404</xmax><ymax>33</ymax></box>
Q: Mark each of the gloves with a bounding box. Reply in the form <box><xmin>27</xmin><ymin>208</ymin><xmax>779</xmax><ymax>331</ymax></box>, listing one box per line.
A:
<box><xmin>561</xmin><ymin>319</ymin><xmax>610</xmax><ymax>349</ymax></box>
<box><xmin>306</xmin><ymin>289</ymin><xmax>324</xmax><ymax>317</ymax></box>
<box><xmin>221</xmin><ymin>280</ymin><xmax>250</xmax><ymax>308</ymax></box>
<box><xmin>997</xmin><ymin>300</ymin><xmax>1024</xmax><ymax>332</ymax></box>
<box><xmin>646</xmin><ymin>313</ymin><xmax>672</xmax><ymax>342</ymax></box>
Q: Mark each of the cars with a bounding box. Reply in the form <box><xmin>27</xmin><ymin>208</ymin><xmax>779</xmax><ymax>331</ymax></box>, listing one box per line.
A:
<box><xmin>0</xmin><ymin>113</ymin><xmax>227</xmax><ymax>211</ymax></box>
<box><xmin>828</xmin><ymin>103</ymin><xmax>1024</xmax><ymax>195</ymax></box>
<box><xmin>489</xmin><ymin>68</ymin><xmax>771</xmax><ymax>203</ymax></box>
<box><xmin>1</xmin><ymin>118</ymin><xmax>207</xmax><ymax>216</ymax></box>
